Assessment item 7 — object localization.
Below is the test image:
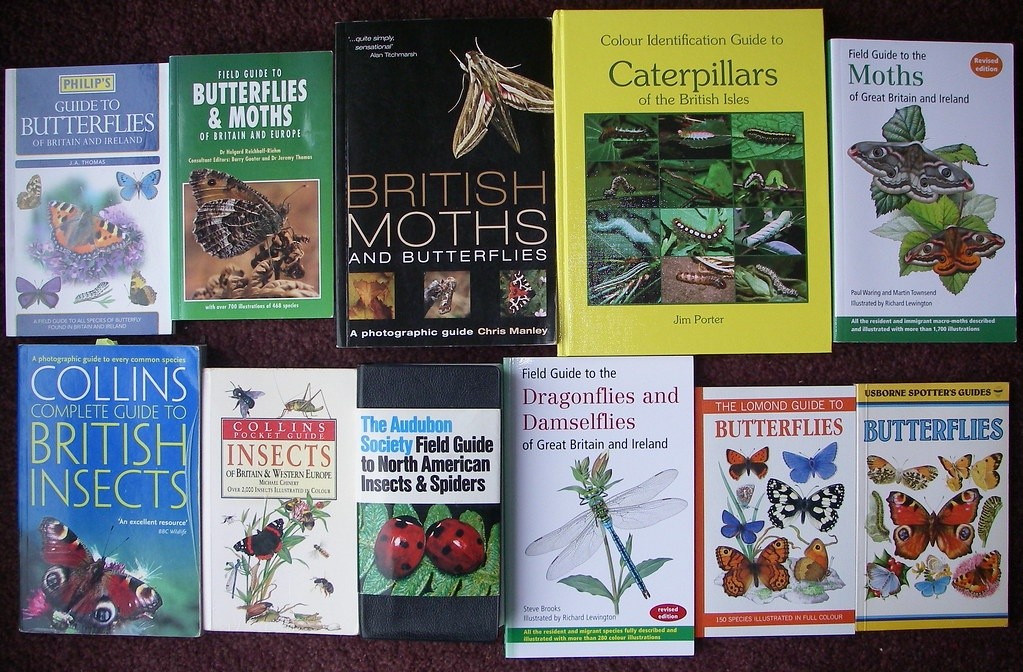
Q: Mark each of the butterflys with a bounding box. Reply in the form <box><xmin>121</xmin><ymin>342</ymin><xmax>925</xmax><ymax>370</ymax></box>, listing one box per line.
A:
<box><xmin>116</xmin><ymin>169</ymin><xmax>161</xmax><ymax>201</ymax></box>
<box><xmin>17</xmin><ymin>174</ymin><xmax>42</xmax><ymax>210</ymax></box>
<box><xmin>232</xmin><ymin>518</ymin><xmax>284</xmax><ymax>561</ymax></box>
<box><xmin>847</xmin><ymin>135</ymin><xmax>976</xmax><ymax>203</ymax></box>
<box><xmin>74</xmin><ymin>281</ymin><xmax>109</xmax><ymax>304</ymax></box>
<box><xmin>767</xmin><ymin>478</ymin><xmax>844</xmax><ymax>532</ymax></box>
<box><xmin>903</xmin><ymin>217</ymin><xmax>1006</xmax><ymax>277</ymax></box>
<box><xmin>188</xmin><ymin>167</ymin><xmax>308</xmax><ymax>261</ymax></box>
<box><xmin>15</xmin><ymin>276</ymin><xmax>61</xmax><ymax>309</ymax></box>
<box><xmin>726</xmin><ymin>446</ymin><xmax>768</xmax><ymax>481</ymax></box>
<box><xmin>865</xmin><ymin>562</ymin><xmax>901</xmax><ymax>599</ymax></box>
<box><xmin>736</xmin><ymin>483</ymin><xmax>755</xmax><ymax>510</ymax></box>
<box><xmin>937</xmin><ymin>451</ymin><xmax>1003</xmax><ymax>493</ymax></box>
<box><xmin>41</xmin><ymin>516</ymin><xmax>167</xmax><ymax>629</ymax></box>
<box><xmin>45</xmin><ymin>200</ymin><xmax>133</xmax><ymax>261</ymax></box>
<box><xmin>507</xmin><ymin>269</ymin><xmax>536</xmax><ymax>317</ymax></box>
<box><xmin>866</xmin><ymin>454</ymin><xmax>939</xmax><ymax>490</ymax></box>
<box><xmin>914</xmin><ymin>576</ymin><xmax>951</xmax><ymax>597</ymax></box>
<box><xmin>720</xmin><ymin>510</ymin><xmax>765</xmax><ymax>544</ymax></box>
<box><xmin>950</xmin><ymin>550</ymin><xmax>1001</xmax><ymax>597</ymax></box>
<box><xmin>783</xmin><ymin>441</ymin><xmax>838</xmax><ymax>483</ymax></box>
<box><xmin>353</xmin><ymin>279</ymin><xmax>387</xmax><ymax>312</ymax></box>
<box><xmin>123</xmin><ymin>271</ymin><xmax>158</xmax><ymax>307</ymax></box>
<box><xmin>715</xmin><ymin>537</ymin><xmax>791</xmax><ymax>598</ymax></box>
<box><xmin>793</xmin><ymin>538</ymin><xmax>834</xmax><ymax>584</ymax></box>
<box><xmin>886</xmin><ymin>487</ymin><xmax>984</xmax><ymax>560</ymax></box>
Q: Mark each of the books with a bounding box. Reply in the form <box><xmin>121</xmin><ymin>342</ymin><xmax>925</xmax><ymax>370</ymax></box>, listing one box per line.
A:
<box><xmin>502</xmin><ymin>357</ymin><xmax>695</xmax><ymax>655</ymax></box>
<box><xmin>856</xmin><ymin>380</ymin><xmax>1009</xmax><ymax>632</ymax></box>
<box><xmin>353</xmin><ymin>363</ymin><xmax>506</xmax><ymax>642</ymax></box>
<box><xmin>198</xmin><ymin>367</ymin><xmax>361</xmax><ymax>637</ymax></box>
<box><xmin>824</xmin><ymin>38</ymin><xmax>1016</xmax><ymax>344</ymax></box>
<box><xmin>552</xmin><ymin>8</ymin><xmax>832</xmax><ymax>355</ymax></box>
<box><xmin>695</xmin><ymin>385</ymin><xmax>858</xmax><ymax>637</ymax></box>
<box><xmin>168</xmin><ymin>50</ymin><xmax>336</xmax><ymax>320</ymax></box>
<box><xmin>333</xmin><ymin>16</ymin><xmax>560</xmax><ymax>346</ymax></box>
<box><xmin>4</xmin><ymin>62</ymin><xmax>175</xmax><ymax>339</ymax></box>
<box><xmin>17</xmin><ymin>344</ymin><xmax>204</xmax><ymax>639</ymax></box>
